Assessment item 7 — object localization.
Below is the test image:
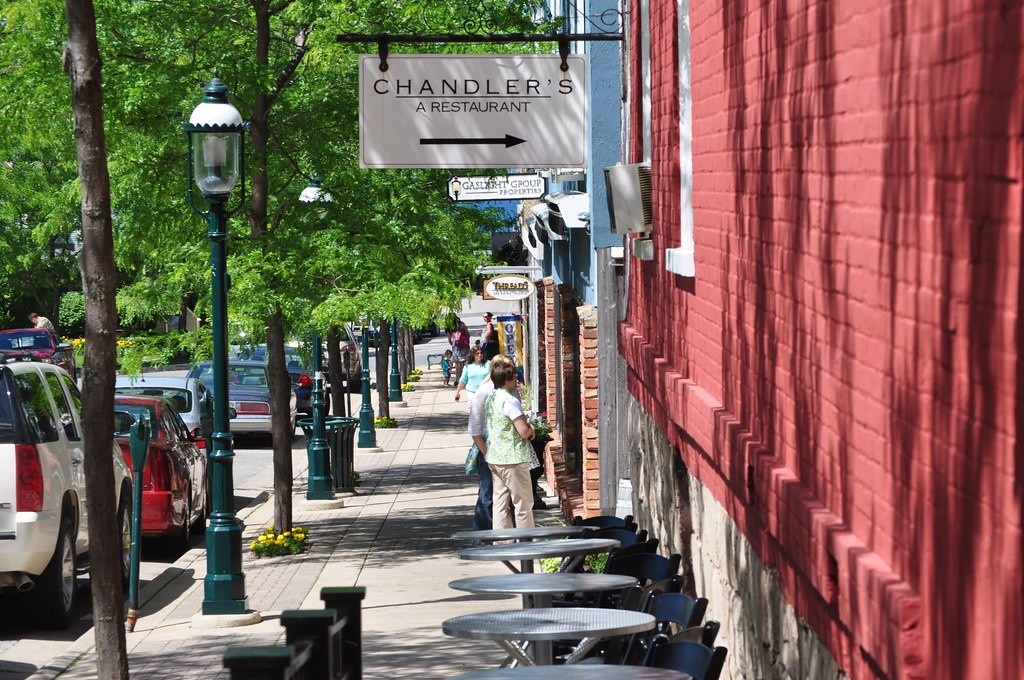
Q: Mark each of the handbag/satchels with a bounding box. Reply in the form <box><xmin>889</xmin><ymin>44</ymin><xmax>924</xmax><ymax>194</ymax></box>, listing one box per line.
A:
<box><xmin>464</xmin><ymin>442</ymin><xmax>480</xmax><ymax>476</ymax></box>
<box><xmin>454</xmin><ymin>322</ymin><xmax>470</xmax><ymax>349</ymax></box>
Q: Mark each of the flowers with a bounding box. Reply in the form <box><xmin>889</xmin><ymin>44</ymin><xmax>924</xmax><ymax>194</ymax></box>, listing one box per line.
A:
<box><xmin>518</xmin><ymin>384</ymin><xmax>553</xmax><ymax>441</ymax></box>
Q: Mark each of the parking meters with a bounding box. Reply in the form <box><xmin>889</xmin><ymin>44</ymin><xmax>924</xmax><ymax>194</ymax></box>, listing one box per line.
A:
<box><xmin>125</xmin><ymin>414</ymin><xmax>154</xmax><ymax>632</ymax></box>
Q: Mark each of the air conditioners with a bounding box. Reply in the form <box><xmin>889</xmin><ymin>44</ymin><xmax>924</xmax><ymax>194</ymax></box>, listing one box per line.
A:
<box><xmin>603</xmin><ymin>160</ymin><xmax>651</xmax><ymax>234</ymax></box>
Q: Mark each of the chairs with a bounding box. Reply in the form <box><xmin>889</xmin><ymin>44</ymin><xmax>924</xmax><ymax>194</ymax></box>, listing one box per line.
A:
<box><xmin>243</xmin><ymin>376</ymin><xmax>262</xmax><ymax>383</ymax></box>
<box><xmin>251</xmin><ymin>355</ymin><xmax>263</xmax><ymax>360</ymax></box>
<box><xmin>34</xmin><ymin>335</ymin><xmax>50</xmax><ymax>345</ymax></box>
<box><xmin>560</xmin><ymin>514</ymin><xmax>727</xmax><ymax>680</ymax></box>
<box><xmin>288</xmin><ymin>361</ymin><xmax>301</xmax><ymax>367</ymax></box>
<box><xmin>0</xmin><ymin>339</ymin><xmax>13</xmax><ymax>348</ymax></box>
<box><xmin>172</xmin><ymin>395</ymin><xmax>187</xmax><ymax>410</ymax></box>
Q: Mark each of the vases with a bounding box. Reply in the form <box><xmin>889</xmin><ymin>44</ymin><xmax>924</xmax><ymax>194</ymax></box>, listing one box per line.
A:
<box><xmin>529</xmin><ymin>436</ymin><xmax>553</xmax><ymax>509</ymax></box>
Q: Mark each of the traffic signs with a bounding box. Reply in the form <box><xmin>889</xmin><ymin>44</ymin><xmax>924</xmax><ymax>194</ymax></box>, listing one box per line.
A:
<box><xmin>354</xmin><ymin>51</ymin><xmax>592</xmax><ymax>176</ymax></box>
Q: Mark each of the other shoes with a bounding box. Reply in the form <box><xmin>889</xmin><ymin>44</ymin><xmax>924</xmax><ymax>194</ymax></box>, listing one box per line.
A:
<box><xmin>454</xmin><ymin>382</ymin><xmax>458</xmax><ymax>388</ymax></box>
<box><xmin>443</xmin><ymin>384</ymin><xmax>452</xmax><ymax>387</ymax></box>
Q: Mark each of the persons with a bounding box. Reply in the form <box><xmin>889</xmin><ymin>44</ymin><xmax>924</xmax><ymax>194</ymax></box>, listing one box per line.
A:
<box><xmin>448</xmin><ymin>312</ymin><xmax>470</xmax><ymax>389</ymax></box>
<box><xmin>442</xmin><ymin>350</ymin><xmax>452</xmax><ymax>386</ymax></box>
<box><xmin>481</xmin><ymin>312</ymin><xmax>494</xmax><ymax>357</ymax></box>
<box><xmin>28</xmin><ymin>313</ymin><xmax>54</xmax><ymax>331</ymax></box>
<box><xmin>453</xmin><ymin>346</ymin><xmax>539</xmax><ymax>549</ymax></box>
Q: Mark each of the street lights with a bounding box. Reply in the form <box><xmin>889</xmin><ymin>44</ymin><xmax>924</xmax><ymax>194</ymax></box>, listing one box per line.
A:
<box><xmin>300</xmin><ymin>187</ymin><xmax>342</xmax><ymax>501</ymax></box>
<box><xmin>179</xmin><ymin>76</ymin><xmax>262</xmax><ymax>612</ymax></box>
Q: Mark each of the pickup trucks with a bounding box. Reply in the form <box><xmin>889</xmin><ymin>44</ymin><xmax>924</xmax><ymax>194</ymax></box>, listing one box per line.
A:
<box><xmin>0</xmin><ymin>329</ymin><xmax>76</xmax><ymax>389</ymax></box>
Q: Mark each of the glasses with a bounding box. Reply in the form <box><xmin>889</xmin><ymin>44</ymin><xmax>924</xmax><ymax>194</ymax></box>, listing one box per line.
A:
<box><xmin>474</xmin><ymin>352</ymin><xmax>484</xmax><ymax>355</ymax></box>
<box><xmin>483</xmin><ymin>316</ymin><xmax>490</xmax><ymax>318</ymax></box>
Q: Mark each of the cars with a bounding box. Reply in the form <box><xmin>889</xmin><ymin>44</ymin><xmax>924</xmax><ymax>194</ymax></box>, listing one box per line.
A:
<box><xmin>114</xmin><ymin>395</ymin><xmax>208</xmax><ymax>548</ymax></box>
<box><xmin>114</xmin><ymin>375</ymin><xmax>237</xmax><ymax>459</ymax></box>
<box><xmin>236</xmin><ymin>346</ymin><xmax>330</xmax><ymax>417</ymax></box>
<box><xmin>362</xmin><ymin>316</ymin><xmax>442</xmax><ymax>348</ymax></box>
<box><xmin>291</xmin><ymin>322</ymin><xmax>363</xmax><ymax>393</ymax></box>
<box><xmin>186</xmin><ymin>360</ymin><xmax>297</xmax><ymax>441</ymax></box>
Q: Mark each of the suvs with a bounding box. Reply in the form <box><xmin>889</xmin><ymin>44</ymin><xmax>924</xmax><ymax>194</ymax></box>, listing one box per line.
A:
<box><xmin>0</xmin><ymin>360</ymin><xmax>134</xmax><ymax>636</ymax></box>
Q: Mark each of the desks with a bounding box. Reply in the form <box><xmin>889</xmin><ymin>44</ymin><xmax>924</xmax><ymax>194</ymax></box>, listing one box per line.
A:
<box><xmin>451</xmin><ymin>525</ymin><xmax>600</xmax><ymax>575</ymax></box>
<box><xmin>457</xmin><ymin>538</ymin><xmax>621</xmax><ymax>607</ymax></box>
<box><xmin>441</xmin><ymin>607</ymin><xmax>656</xmax><ymax>668</ymax></box>
<box><xmin>448</xmin><ymin>573</ymin><xmax>638</xmax><ymax>670</ymax></box>
<box><xmin>464</xmin><ymin>663</ymin><xmax>695</xmax><ymax>680</ymax></box>
